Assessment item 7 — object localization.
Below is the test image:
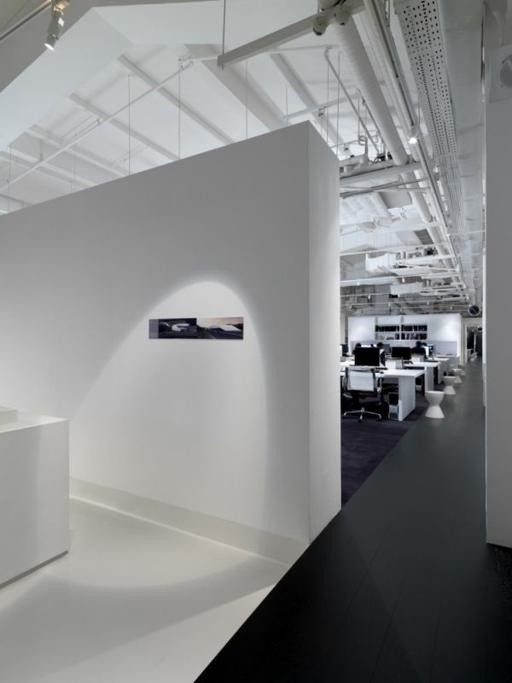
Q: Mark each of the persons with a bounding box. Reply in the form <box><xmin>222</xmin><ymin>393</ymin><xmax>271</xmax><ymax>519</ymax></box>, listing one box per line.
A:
<box><xmin>351</xmin><ymin>342</ymin><xmax>361</xmax><ymax>354</ymax></box>
<box><xmin>410</xmin><ymin>340</ymin><xmax>425</xmax><ymax>354</ymax></box>
<box><xmin>375</xmin><ymin>341</ymin><xmax>386</xmax><ymax>369</ymax></box>
<box><xmin>420</xmin><ymin>342</ymin><xmax>432</xmax><ymax>358</ymax></box>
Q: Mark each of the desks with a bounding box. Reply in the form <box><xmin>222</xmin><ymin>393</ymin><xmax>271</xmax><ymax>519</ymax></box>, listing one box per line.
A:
<box><xmin>340</xmin><ymin>340</ymin><xmax>462</xmax><ymax>422</ymax></box>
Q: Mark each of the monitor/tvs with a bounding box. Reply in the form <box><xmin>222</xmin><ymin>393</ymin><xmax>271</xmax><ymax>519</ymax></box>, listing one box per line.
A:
<box><xmin>354</xmin><ymin>347</ymin><xmax>379</xmax><ymax>366</ymax></box>
<box><xmin>422</xmin><ymin>345</ymin><xmax>429</xmax><ymax>358</ymax></box>
<box><xmin>390</xmin><ymin>346</ymin><xmax>411</xmax><ymax>359</ymax></box>
<box><xmin>362</xmin><ymin>343</ymin><xmax>377</xmax><ymax>347</ymax></box>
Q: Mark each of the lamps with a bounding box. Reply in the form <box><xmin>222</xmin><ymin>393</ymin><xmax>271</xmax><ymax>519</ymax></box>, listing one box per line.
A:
<box><xmin>42</xmin><ymin>0</ymin><xmax>72</xmax><ymax>52</ymax></box>
